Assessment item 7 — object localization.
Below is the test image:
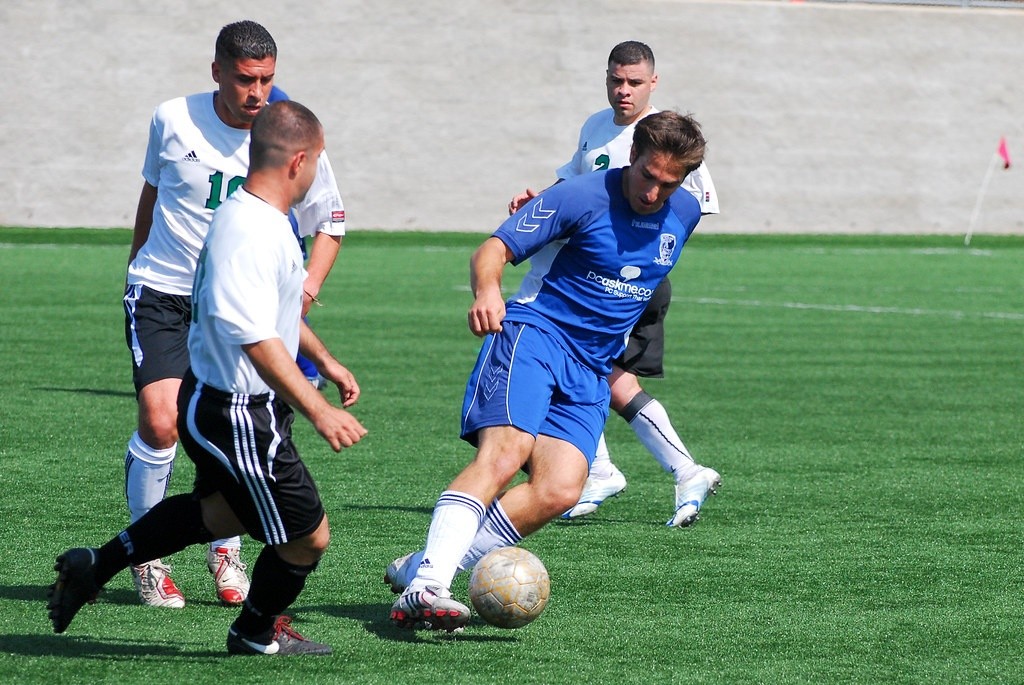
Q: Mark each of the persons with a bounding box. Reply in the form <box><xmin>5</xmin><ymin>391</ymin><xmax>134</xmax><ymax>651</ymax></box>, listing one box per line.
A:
<box><xmin>120</xmin><ymin>20</ymin><xmax>349</xmax><ymax>609</ymax></box>
<box><xmin>384</xmin><ymin>111</ymin><xmax>707</xmax><ymax>630</ymax></box>
<box><xmin>47</xmin><ymin>101</ymin><xmax>369</xmax><ymax>658</ymax></box>
<box><xmin>508</xmin><ymin>40</ymin><xmax>720</xmax><ymax>530</ymax></box>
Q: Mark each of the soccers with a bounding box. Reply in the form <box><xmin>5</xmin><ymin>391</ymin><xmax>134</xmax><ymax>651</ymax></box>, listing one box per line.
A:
<box><xmin>467</xmin><ymin>545</ymin><xmax>551</xmax><ymax>630</ymax></box>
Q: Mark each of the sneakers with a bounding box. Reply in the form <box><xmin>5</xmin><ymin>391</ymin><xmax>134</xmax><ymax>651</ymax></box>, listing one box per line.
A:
<box><xmin>389</xmin><ymin>581</ymin><xmax>472</xmax><ymax>634</ymax></box>
<box><xmin>45</xmin><ymin>548</ymin><xmax>98</xmax><ymax>634</ymax></box>
<box><xmin>130</xmin><ymin>563</ymin><xmax>185</xmax><ymax>609</ymax></box>
<box><xmin>383</xmin><ymin>553</ymin><xmax>417</xmax><ymax>594</ymax></box>
<box><xmin>206</xmin><ymin>547</ymin><xmax>251</xmax><ymax>604</ymax></box>
<box><xmin>560</xmin><ymin>464</ymin><xmax>627</xmax><ymax>521</ymax></box>
<box><xmin>667</xmin><ymin>465</ymin><xmax>721</xmax><ymax>528</ymax></box>
<box><xmin>226</xmin><ymin>615</ymin><xmax>331</xmax><ymax>657</ymax></box>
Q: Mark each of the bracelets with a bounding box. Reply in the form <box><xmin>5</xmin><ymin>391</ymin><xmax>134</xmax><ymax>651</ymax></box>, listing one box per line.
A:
<box><xmin>303</xmin><ymin>289</ymin><xmax>323</xmax><ymax>307</ymax></box>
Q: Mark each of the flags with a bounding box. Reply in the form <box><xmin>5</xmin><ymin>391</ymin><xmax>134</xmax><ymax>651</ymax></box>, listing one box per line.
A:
<box><xmin>997</xmin><ymin>137</ymin><xmax>1011</xmax><ymax>167</ymax></box>
